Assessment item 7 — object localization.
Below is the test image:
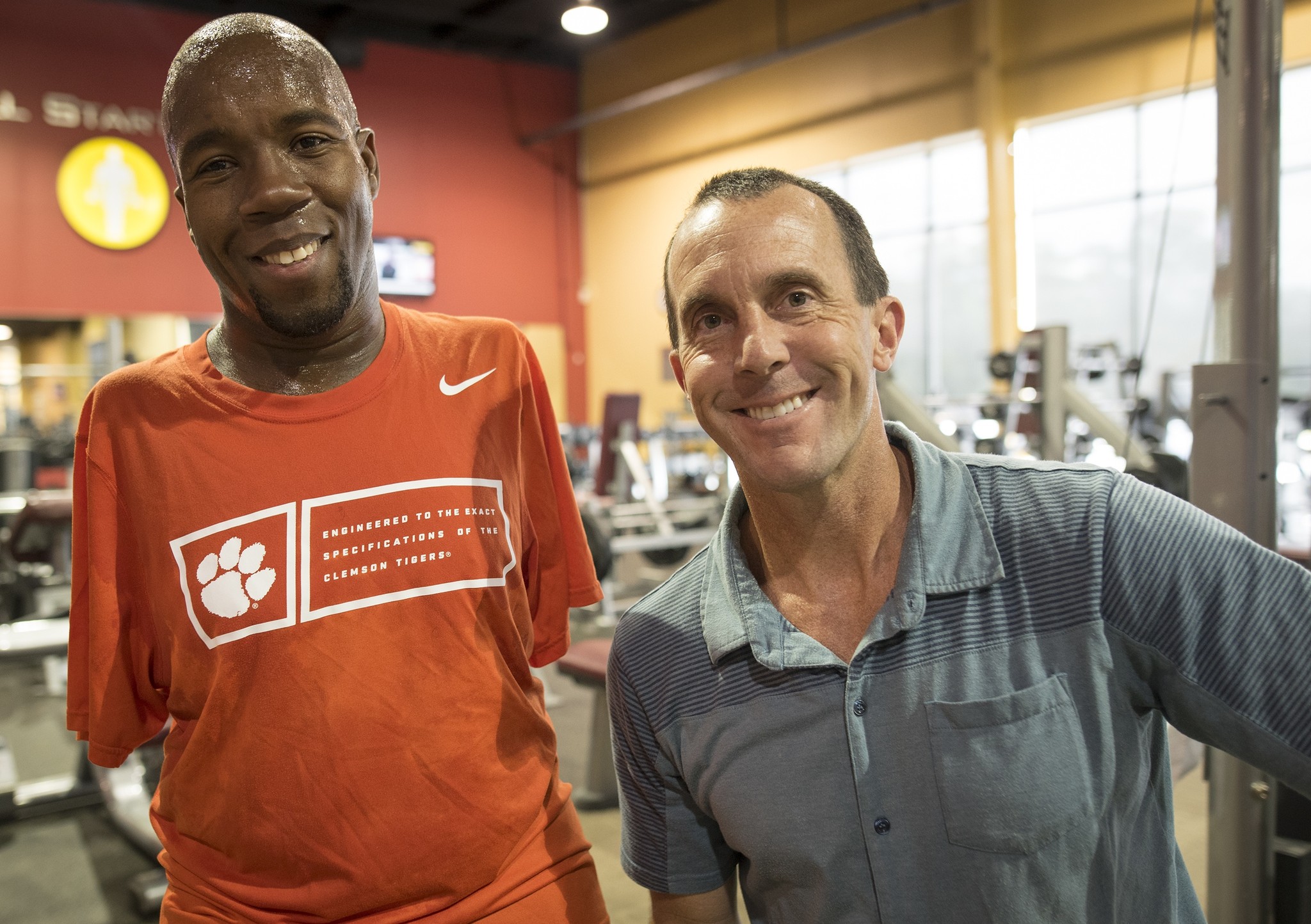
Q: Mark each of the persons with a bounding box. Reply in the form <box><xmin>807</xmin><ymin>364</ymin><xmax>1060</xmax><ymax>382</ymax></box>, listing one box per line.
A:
<box><xmin>67</xmin><ymin>24</ymin><xmax>618</xmax><ymax>924</ymax></box>
<box><xmin>603</xmin><ymin>167</ymin><xmax>1311</xmax><ymax>924</ymax></box>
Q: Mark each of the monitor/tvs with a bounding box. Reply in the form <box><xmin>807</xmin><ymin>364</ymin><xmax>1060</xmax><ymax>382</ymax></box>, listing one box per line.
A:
<box><xmin>594</xmin><ymin>392</ymin><xmax>640</xmax><ymax>504</ymax></box>
<box><xmin>371</xmin><ymin>236</ymin><xmax>438</xmax><ymax>297</ymax></box>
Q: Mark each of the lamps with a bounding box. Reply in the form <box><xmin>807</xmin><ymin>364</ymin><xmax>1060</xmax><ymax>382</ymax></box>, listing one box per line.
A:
<box><xmin>561</xmin><ymin>0</ymin><xmax>609</xmax><ymax>36</ymax></box>
